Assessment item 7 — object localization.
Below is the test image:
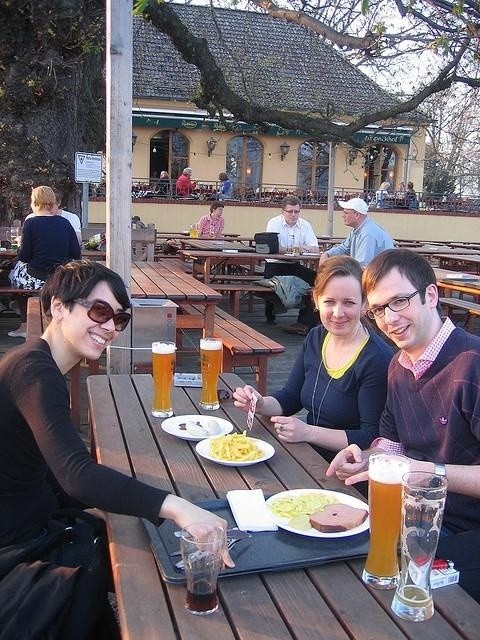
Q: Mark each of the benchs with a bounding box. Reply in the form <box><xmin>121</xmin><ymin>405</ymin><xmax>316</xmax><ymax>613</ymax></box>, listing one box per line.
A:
<box><xmin>179</xmin><ymin>299</ymin><xmax>291</xmax><ymax>397</ymax></box>
<box><xmin>21</xmin><ymin>296</ymin><xmax>102</xmax><ymax>424</ymax></box>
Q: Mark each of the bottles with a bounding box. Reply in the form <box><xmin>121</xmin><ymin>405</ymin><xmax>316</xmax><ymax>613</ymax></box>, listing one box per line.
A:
<box><xmin>287</xmin><ymin>234</ymin><xmax>295</xmax><ymax>253</ymax></box>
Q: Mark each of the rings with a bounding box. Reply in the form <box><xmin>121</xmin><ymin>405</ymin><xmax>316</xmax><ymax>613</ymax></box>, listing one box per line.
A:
<box><xmin>280</xmin><ymin>424</ymin><xmax>284</xmax><ymax>431</ymax></box>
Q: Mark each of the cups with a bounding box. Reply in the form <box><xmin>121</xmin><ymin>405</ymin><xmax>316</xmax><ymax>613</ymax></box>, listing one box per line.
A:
<box><xmin>197</xmin><ymin>337</ymin><xmax>224</xmax><ymax>412</ymax></box>
<box><xmin>152</xmin><ymin>340</ymin><xmax>174</xmax><ymax>420</ymax></box>
<box><xmin>180</xmin><ymin>522</ymin><xmax>227</xmax><ymax>613</ymax></box>
<box><xmin>189</xmin><ymin>223</ymin><xmax>198</xmax><ymax>238</ymax></box>
<box><xmin>390</xmin><ymin>472</ymin><xmax>449</xmax><ymax>621</ymax></box>
<box><xmin>361</xmin><ymin>450</ymin><xmax>414</xmax><ymax>591</ymax></box>
<box><xmin>5</xmin><ymin>227</ymin><xmax>19</xmax><ymax>252</ymax></box>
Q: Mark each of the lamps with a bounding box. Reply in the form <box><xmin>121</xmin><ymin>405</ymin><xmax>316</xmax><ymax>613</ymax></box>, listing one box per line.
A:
<box><xmin>206</xmin><ymin>137</ymin><xmax>218</xmax><ymax>157</ymax></box>
<box><xmin>280</xmin><ymin>141</ymin><xmax>291</xmax><ymax>161</ymax></box>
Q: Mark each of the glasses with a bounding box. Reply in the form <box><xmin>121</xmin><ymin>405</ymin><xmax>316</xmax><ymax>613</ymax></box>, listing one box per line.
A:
<box><xmin>74</xmin><ymin>297</ymin><xmax>131</xmax><ymax>332</ymax></box>
<box><xmin>283</xmin><ymin>210</ymin><xmax>300</xmax><ymax>213</ymax></box>
<box><xmin>367</xmin><ymin>287</ymin><xmax>422</xmax><ymax>320</ymax></box>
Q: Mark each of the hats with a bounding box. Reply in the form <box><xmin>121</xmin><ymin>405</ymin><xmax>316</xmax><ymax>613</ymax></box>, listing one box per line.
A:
<box><xmin>338</xmin><ymin>198</ymin><xmax>368</xmax><ymax>216</ymax></box>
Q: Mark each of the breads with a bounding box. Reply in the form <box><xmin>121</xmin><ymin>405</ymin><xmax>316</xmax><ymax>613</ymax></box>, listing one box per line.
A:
<box><xmin>309</xmin><ymin>503</ymin><xmax>369</xmax><ymax>533</ymax></box>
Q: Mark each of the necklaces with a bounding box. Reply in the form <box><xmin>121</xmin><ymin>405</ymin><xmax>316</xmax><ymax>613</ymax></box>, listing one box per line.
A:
<box><xmin>312</xmin><ymin>356</ymin><xmax>337</xmax><ymax>426</ymax></box>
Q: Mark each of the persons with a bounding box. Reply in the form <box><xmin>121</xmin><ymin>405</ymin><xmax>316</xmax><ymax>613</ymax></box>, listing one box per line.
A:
<box><xmin>376</xmin><ymin>182</ymin><xmax>390</xmax><ymax>208</ymax></box>
<box><xmin>0</xmin><ymin>260</ymin><xmax>236</xmax><ymax>640</ymax></box>
<box><xmin>403</xmin><ymin>182</ymin><xmax>416</xmax><ymax>207</ymax></box>
<box><xmin>233</xmin><ymin>255</ymin><xmax>395</xmax><ymax>499</ymax></box>
<box><xmin>279</xmin><ymin>198</ymin><xmax>395</xmax><ymax>335</ymax></box>
<box><xmin>264</xmin><ymin>195</ymin><xmax>320</xmax><ymax>324</ymax></box>
<box><xmin>195</xmin><ymin>202</ymin><xmax>224</xmax><ymax>236</ymax></box>
<box><xmin>152</xmin><ymin>171</ymin><xmax>169</xmax><ymax>195</ymax></box>
<box><xmin>176</xmin><ymin>168</ymin><xmax>196</xmax><ymax>197</ymax></box>
<box><xmin>8</xmin><ymin>186</ymin><xmax>81</xmax><ymax>338</ymax></box>
<box><xmin>25</xmin><ymin>187</ymin><xmax>82</xmax><ymax>247</ymax></box>
<box><xmin>208</xmin><ymin>173</ymin><xmax>233</xmax><ymax>200</ymax></box>
<box><xmin>327</xmin><ymin>248</ymin><xmax>480</xmax><ymax>546</ymax></box>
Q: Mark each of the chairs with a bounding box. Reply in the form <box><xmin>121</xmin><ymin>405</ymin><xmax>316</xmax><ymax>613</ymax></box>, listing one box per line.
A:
<box><xmin>98</xmin><ymin>180</ymin><xmax>476</xmax><ymax>214</ymax></box>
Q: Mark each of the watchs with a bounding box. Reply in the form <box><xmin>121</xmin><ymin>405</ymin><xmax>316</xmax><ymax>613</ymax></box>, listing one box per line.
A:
<box><xmin>430</xmin><ymin>463</ymin><xmax>445</xmax><ymax>486</ymax></box>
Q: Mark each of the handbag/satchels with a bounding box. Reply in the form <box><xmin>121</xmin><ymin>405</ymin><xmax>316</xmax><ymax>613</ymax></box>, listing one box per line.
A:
<box><xmin>254</xmin><ymin>233</ymin><xmax>280</xmax><ymax>254</ymax></box>
<box><xmin>43</xmin><ymin>510</ymin><xmax>113</xmax><ymax>590</ymax></box>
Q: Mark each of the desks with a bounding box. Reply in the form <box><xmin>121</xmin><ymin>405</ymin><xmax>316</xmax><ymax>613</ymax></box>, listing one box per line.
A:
<box><xmin>82</xmin><ymin>369</ymin><xmax>479</xmax><ymax>640</ymax></box>
<box><xmin>1</xmin><ymin>230</ymin><xmax>479</xmax><ymax>338</ymax></box>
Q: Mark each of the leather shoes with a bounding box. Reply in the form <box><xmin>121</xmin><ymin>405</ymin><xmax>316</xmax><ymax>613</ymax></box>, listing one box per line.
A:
<box><xmin>9</xmin><ymin>301</ymin><xmax>21</xmax><ymax>314</ymax></box>
<box><xmin>268</xmin><ymin>316</ymin><xmax>280</xmax><ymax>325</ymax></box>
<box><xmin>8</xmin><ymin>331</ymin><xmax>27</xmax><ymax>339</ymax></box>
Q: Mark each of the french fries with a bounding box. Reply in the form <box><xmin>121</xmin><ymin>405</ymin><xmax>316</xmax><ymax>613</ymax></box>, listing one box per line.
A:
<box><xmin>211</xmin><ymin>430</ymin><xmax>263</xmax><ymax>464</ymax></box>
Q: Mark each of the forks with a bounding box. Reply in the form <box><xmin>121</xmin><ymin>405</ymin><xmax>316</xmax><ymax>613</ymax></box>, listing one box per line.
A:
<box><xmin>168</xmin><ymin>527</ymin><xmax>252</xmax><ymax>572</ymax></box>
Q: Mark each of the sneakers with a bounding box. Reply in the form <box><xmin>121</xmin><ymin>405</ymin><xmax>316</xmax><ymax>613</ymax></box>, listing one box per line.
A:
<box><xmin>279</xmin><ymin>323</ymin><xmax>309</xmax><ymax>335</ymax></box>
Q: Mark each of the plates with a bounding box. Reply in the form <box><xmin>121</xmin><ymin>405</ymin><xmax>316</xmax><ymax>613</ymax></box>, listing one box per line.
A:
<box><xmin>194</xmin><ymin>434</ymin><xmax>277</xmax><ymax>465</ymax></box>
<box><xmin>160</xmin><ymin>414</ymin><xmax>233</xmax><ymax>441</ymax></box>
<box><xmin>265</xmin><ymin>487</ymin><xmax>371</xmax><ymax>539</ymax></box>
